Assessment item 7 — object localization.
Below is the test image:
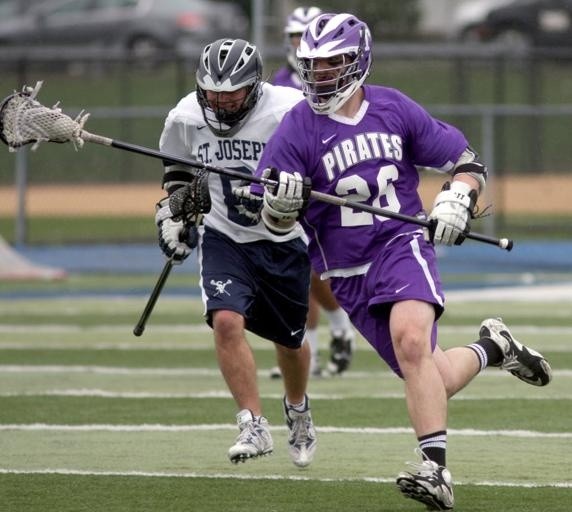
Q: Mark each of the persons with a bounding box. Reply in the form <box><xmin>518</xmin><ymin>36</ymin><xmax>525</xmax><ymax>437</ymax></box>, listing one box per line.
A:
<box><xmin>155</xmin><ymin>38</ymin><xmax>317</xmax><ymax>469</ymax></box>
<box><xmin>269</xmin><ymin>5</ymin><xmax>356</xmax><ymax>379</ymax></box>
<box><xmin>250</xmin><ymin>12</ymin><xmax>552</xmax><ymax>510</ymax></box>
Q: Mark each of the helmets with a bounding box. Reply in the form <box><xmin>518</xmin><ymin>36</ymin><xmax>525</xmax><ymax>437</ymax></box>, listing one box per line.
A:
<box><xmin>195</xmin><ymin>38</ymin><xmax>262</xmax><ymax>137</ymax></box>
<box><xmin>285</xmin><ymin>6</ymin><xmax>373</xmax><ymax>115</ymax></box>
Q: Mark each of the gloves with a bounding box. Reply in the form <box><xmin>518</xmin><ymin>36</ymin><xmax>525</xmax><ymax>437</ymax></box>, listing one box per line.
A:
<box><xmin>155</xmin><ymin>197</ymin><xmax>203</xmax><ymax>261</ymax></box>
<box><xmin>423</xmin><ymin>182</ymin><xmax>491</xmax><ymax>245</ymax></box>
<box><xmin>232</xmin><ymin>168</ymin><xmax>312</xmax><ymax>235</ymax></box>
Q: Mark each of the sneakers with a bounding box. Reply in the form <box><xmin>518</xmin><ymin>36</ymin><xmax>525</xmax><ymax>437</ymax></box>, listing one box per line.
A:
<box><xmin>228</xmin><ymin>409</ymin><xmax>272</xmax><ymax>464</ymax></box>
<box><xmin>283</xmin><ymin>394</ymin><xmax>317</xmax><ymax>467</ymax></box>
<box><xmin>327</xmin><ymin>329</ymin><xmax>355</xmax><ymax>372</ymax></box>
<box><xmin>397</xmin><ymin>447</ymin><xmax>454</xmax><ymax>510</ymax></box>
<box><xmin>478</xmin><ymin>318</ymin><xmax>551</xmax><ymax>386</ymax></box>
